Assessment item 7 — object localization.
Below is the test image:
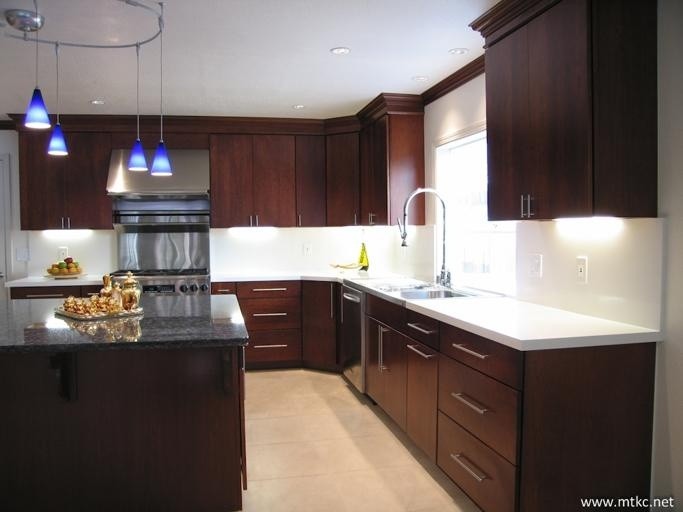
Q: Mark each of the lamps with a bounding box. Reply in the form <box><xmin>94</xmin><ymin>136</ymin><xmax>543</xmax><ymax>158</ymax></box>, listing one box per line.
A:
<box><xmin>0</xmin><ymin>0</ymin><xmax>173</xmax><ymax>177</ymax></box>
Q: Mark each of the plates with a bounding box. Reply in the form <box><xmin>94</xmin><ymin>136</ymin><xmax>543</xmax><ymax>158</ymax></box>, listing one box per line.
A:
<box><xmin>53</xmin><ymin>314</ymin><xmax>145</xmax><ymax>326</ymax></box>
<box><xmin>43</xmin><ymin>275</ymin><xmax>86</xmax><ymax>280</ymax></box>
<box><xmin>54</xmin><ymin>304</ymin><xmax>147</xmax><ymax>320</ymax></box>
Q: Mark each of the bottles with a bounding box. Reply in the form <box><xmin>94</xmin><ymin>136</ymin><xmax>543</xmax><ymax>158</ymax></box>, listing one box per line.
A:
<box><xmin>65</xmin><ymin>321</ymin><xmax>143</xmax><ymax>343</ymax></box>
<box><xmin>358</xmin><ymin>243</ymin><xmax>369</xmax><ymax>273</ymax></box>
<box><xmin>63</xmin><ymin>271</ymin><xmax>141</xmax><ymax>314</ymax></box>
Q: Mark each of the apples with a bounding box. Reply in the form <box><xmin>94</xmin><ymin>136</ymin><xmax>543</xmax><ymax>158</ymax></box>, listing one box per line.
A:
<box><xmin>47</xmin><ymin>262</ymin><xmax>81</xmax><ymax>273</ymax></box>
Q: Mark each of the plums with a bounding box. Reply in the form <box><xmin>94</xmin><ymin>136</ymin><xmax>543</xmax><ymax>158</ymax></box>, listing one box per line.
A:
<box><xmin>64</xmin><ymin>257</ymin><xmax>72</xmax><ymax>263</ymax></box>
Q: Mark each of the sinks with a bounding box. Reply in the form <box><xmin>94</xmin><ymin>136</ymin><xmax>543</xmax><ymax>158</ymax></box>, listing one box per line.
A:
<box><xmin>383</xmin><ymin>289</ymin><xmax>467</xmax><ymax>301</ymax></box>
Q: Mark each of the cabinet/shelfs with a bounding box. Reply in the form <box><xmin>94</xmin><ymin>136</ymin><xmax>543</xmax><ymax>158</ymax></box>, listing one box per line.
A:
<box><xmin>237</xmin><ymin>282</ymin><xmax>302</xmax><ymax>363</ymax></box>
<box><xmin>3</xmin><ymin>110</ymin><xmax>113</xmax><ymax>231</ymax></box>
<box><xmin>210</xmin><ymin>116</ymin><xmax>296</xmax><ymax>228</ymax></box>
<box><xmin>297</xmin><ymin>91</ymin><xmax>425</xmax><ymax>227</ymax></box>
<box><xmin>302</xmin><ymin>281</ymin><xmax>337</xmax><ymax>370</ymax></box>
<box><xmin>339</xmin><ymin>283</ymin><xmax>366</xmax><ymax>393</ymax></box>
<box><xmin>468</xmin><ymin>0</ymin><xmax>658</xmax><ymax>221</ymax></box>
<box><xmin>438</xmin><ymin>322</ymin><xmax>656</xmax><ymax>512</ymax></box>
<box><xmin>407</xmin><ymin>309</ymin><xmax>439</xmax><ymax>466</ymax></box>
<box><xmin>211</xmin><ymin>281</ymin><xmax>235</xmax><ymax>294</ymax></box>
<box><xmin>365</xmin><ymin>292</ymin><xmax>406</xmax><ymax>434</ymax></box>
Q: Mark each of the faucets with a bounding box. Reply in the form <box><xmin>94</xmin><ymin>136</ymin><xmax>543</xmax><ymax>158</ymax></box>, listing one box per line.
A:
<box><xmin>398</xmin><ymin>188</ymin><xmax>446</xmax><ymax>282</ymax></box>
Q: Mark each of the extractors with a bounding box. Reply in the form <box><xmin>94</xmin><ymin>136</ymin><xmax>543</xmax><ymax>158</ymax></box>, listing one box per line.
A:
<box><xmin>105</xmin><ymin>149</ymin><xmax>209</xmax><ymax>197</ymax></box>
